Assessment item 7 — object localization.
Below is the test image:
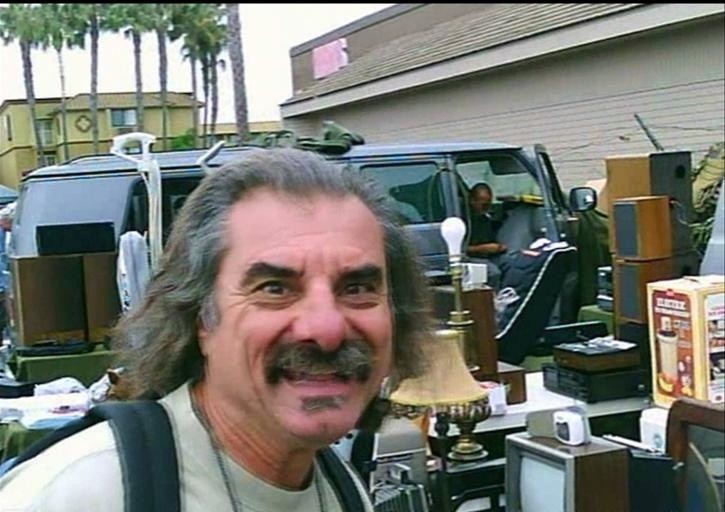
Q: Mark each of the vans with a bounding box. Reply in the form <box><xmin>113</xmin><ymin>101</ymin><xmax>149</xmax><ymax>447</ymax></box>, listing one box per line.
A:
<box><xmin>9</xmin><ymin>139</ymin><xmax>608</xmax><ymax>389</ymax></box>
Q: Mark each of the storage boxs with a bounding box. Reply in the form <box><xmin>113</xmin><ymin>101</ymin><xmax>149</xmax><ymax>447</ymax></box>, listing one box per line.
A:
<box><xmin>646</xmin><ymin>274</ymin><xmax>725</xmax><ymax>408</ymax></box>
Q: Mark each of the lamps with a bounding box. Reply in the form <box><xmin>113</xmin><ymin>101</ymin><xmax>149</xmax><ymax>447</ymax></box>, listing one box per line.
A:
<box><xmin>389</xmin><ymin>330</ymin><xmax>488</xmax><ymax>512</ymax></box>
<box><xmin>439</xmin><ymin>216</ymin><xmax>481</xmax><ymax>373</ymax></box>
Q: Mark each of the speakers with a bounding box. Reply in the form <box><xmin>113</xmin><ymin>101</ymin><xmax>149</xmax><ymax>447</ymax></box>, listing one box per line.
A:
<box><xmin>610</xmin><ymin>197</ymin><xmax>673</xmax><ymax>263</ymax></box>
<box><xmin>609</xmin><ymin>261</ymin><xmax>675</xmax><ymax>326</ymax></box>
<box><xmin>606</xmin><ymin>150</ymin><xmax>693</xmax><ymax>259</ymax></box>
<box><xmin>675</xmin><ymin>252</ymin><xmax>698</xmax><ymax>283</ymax></box>
<box><xmin>426</xmin><ymin>285</ymin><xmax>500</xmax><ymax>377</ymax></box>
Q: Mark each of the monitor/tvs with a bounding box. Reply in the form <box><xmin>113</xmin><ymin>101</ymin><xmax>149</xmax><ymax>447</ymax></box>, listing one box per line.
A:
<box><xmin>506</xmin><ymin>429</ymin><xmax>632</xmax><ymax>511</ymax></box>
<box><xmin>666</xmin><ymin>396</ymin><xmax>725</xmax><ymax>512</ymax></box>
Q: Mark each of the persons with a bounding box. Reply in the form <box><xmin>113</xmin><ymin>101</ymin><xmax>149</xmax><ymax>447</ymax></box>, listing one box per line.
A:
<box><xmin>0</xmin><ymin>147</ymin><xmax>435</xmax><ymax>511</ymax></box>
<box><xmin>461</xmin><ymin>182</ymin><xmax>508</xmax><ymax>295</ymax></box>
<box><xmin>0</xmin><ymin>204</ymin><xmax>17</xmax><ymax>328</ymax></box>
